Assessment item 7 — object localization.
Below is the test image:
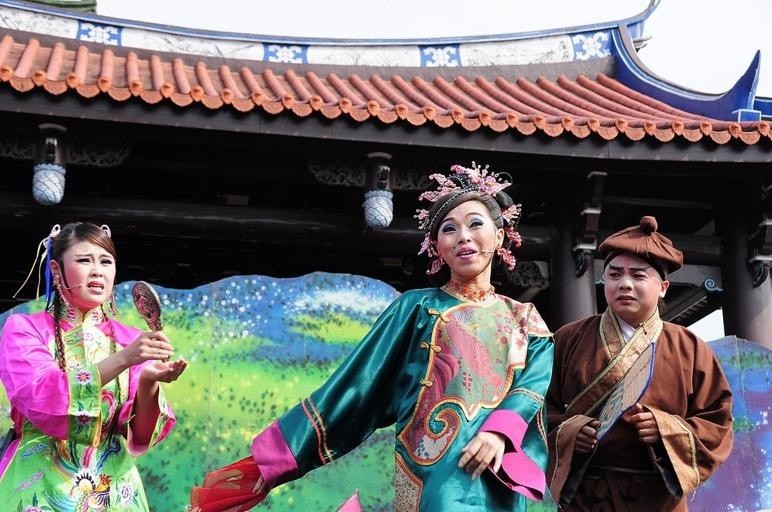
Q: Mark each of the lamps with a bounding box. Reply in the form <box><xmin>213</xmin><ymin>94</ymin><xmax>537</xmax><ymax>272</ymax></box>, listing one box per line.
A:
<box><xmin>31</xmin><ymin>121</ymin><xmax>68</xmax><ymax>206</ymax></box>
<box><xmin>361</xmin><ymin>152</ymin><xmax>397</xmax><ymax>229</ymax></box>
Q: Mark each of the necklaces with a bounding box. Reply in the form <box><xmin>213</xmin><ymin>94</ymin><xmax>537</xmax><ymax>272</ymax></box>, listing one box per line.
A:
<box><xmin>447</xmin><ymin>277</ymin><xmax>496</xmax><ymax>304</ymax></box>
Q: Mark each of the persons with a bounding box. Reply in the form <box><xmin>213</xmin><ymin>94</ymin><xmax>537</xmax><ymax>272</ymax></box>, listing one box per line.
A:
<box><xmin>1</xmin><ymin>217</ymin><xmax>190</xmax><ymax>512</ymax></box>
<box><xmin>539</xmin><ymin>214</ymin><xmax>737</xmax><ymax>512</ymax></box>
<box><xmin>249</xmin><ymin>161</ymin><xmax>565</xmax><ymax>511</ymax></box>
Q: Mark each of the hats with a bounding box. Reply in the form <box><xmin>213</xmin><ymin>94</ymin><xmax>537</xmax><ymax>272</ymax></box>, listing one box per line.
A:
<box><xmin>598</xmin><ymin>215</ymin><xmax>683</xmax><ymax>281</ymax></box>
